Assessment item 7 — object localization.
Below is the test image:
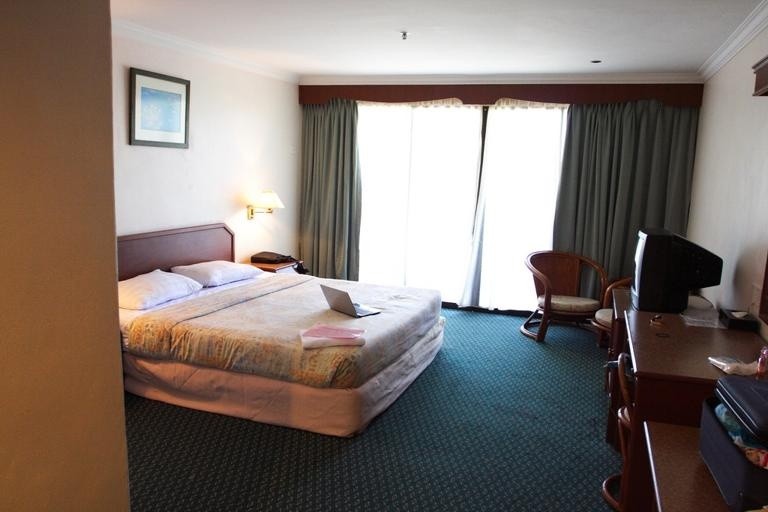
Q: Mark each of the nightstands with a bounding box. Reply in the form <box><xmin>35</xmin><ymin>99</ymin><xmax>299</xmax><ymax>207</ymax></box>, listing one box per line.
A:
<box><xmin>239</xmin><ymin>258</ymin><xmax>304</xmax><ymax>273</ymax></box>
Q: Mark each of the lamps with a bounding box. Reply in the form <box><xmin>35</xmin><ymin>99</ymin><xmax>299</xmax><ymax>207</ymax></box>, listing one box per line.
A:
<box><xmin>247</xmin><ymin>189</ymin><xmax>285</xmax><ymax>220</ymax></box>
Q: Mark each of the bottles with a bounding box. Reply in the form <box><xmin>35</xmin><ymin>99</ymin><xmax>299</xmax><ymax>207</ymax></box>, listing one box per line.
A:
<box><xmin>757</xmin><ymin>346</ymin><xmax>768</xmax><ymax>376</ymax></box>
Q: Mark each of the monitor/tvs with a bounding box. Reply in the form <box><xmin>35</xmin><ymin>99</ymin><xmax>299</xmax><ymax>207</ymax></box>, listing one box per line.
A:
<box><xmin>630</xmin><ymin>227</ymin><xmax>723</xmax><ymax>312</ymax></box>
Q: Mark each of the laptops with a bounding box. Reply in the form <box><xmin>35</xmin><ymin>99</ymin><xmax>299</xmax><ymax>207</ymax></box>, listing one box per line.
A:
<box><xmin>320</xmin><ymin>283</ymin><xmax>382</xmax><ymax>319</ymax></box>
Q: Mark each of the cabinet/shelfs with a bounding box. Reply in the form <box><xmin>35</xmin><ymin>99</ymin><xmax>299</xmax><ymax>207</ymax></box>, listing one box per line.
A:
<box><xmin>601</xmin><ymin>287</ymin><xmax>768</xmax><ymax>512</ymax></box>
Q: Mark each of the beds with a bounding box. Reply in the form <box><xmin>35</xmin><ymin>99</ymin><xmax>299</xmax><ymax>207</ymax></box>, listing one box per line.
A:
<box><xmin>117</xmin><ymin>223</ymin><xmax>446</xmax><ymax>437</ymax></box>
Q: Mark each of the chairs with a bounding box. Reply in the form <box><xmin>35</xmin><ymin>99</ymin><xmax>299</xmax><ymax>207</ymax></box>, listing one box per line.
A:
<box><xmin>590</xmin><ymin>277</ymin><xmax>633</xmax><ymax>347</ymax></box>
<box><xmin>519</xmin><ymin>251</ymin><xmax>608</xmax><ymax>343</ymax></box>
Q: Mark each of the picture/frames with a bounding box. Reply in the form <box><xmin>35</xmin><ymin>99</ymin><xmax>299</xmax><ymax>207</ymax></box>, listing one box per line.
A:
<box><xmin>129</xmin><ymin>67</ymin><xmax>190</xmax><ymax>149</ymax></box>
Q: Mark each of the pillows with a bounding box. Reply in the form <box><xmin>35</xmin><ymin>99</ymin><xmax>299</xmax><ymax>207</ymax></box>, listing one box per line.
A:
<box><xmin>170</xmin><ymin>260</ymin><xmax>264</xmax><ymax>287</ymax></box>
<box><xmin>117</xmin><ymin>268</ymin><xmax>204</xmax><ymax>310</ymax></box>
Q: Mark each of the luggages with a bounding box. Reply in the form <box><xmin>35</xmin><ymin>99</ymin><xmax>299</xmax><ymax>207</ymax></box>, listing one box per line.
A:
<box><xmin>695</xmin><ymin>376</ymin><xmax>768</xmax><ymax>512</ymax></box>
